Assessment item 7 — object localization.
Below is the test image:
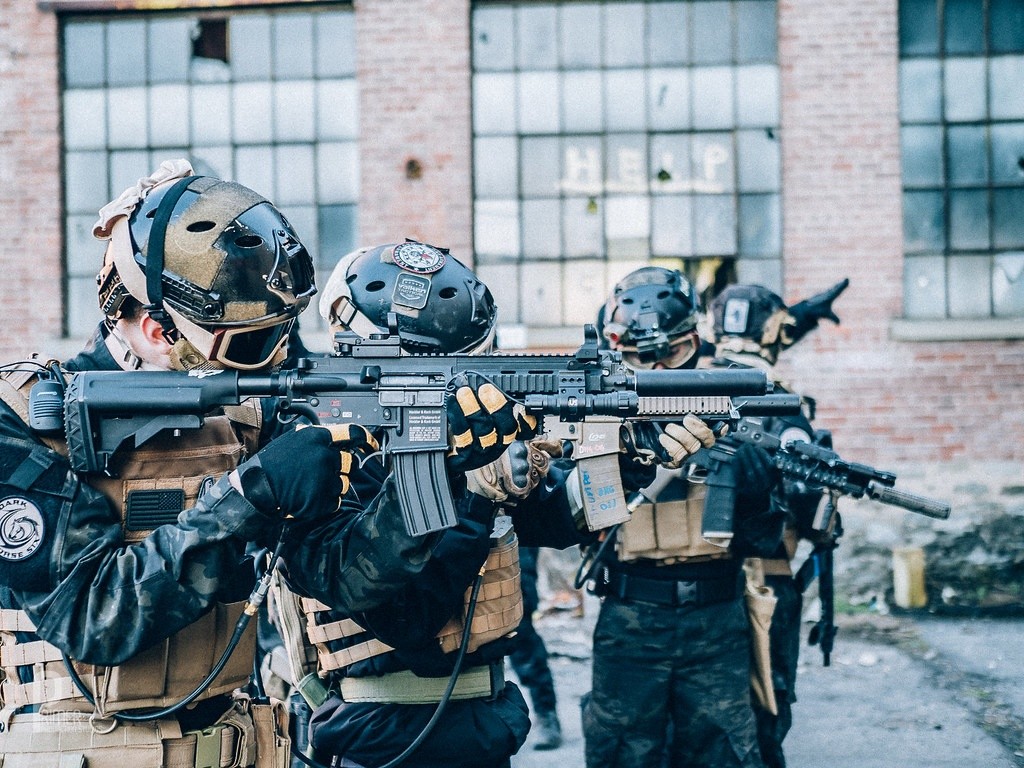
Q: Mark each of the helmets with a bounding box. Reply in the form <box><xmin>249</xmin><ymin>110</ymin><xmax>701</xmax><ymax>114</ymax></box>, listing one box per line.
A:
<box><xmin>601</xmin><ymin>267</ymin><xmax>700</xmax><ymax>348</ymax></box>
<box><xmin>323</xmin><ymin>238</ymin><xmax>499</xmax><ymax>362</ymax></box>
<box><xmin>711</xmin><ymin>283</ymin><xmax>790</xmax><ymax>345</ymax></box>
<box><xmin>95</xmin><ymin>175</ymin><xmax>317</xmax><ymax>332</ymax></box>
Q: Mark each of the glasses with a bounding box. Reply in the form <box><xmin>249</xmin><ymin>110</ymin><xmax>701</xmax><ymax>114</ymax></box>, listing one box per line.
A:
<box><xmin>616</xmin><ymin>335</ymin><xmax>698</xmax><ymax>372</ymax></box>
<box><xmin>95</xmin><ymin>160</ymin><xmax>295</xmax><ymax>369</ymax></box>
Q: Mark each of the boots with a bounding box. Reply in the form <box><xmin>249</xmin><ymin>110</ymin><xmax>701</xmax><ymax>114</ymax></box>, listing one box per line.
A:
<box><xmin>533</xmin><ymin>702</ymin><xmax>562</xmax><ymax>748</ymax></box>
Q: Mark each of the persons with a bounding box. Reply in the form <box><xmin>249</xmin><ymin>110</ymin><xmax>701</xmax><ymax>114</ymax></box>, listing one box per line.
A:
<box><xmin>503</xmin><ymin>541</ymin><xmax>565</xmax><ymax>751</ymax></box>
<box><xmin>0</xmin><ymin>161</ymin><xmax>538</xmax><ymax>767</ymax></box>
<box><xmin>709</xmin><ymin>273</ymin><xmax>853</xmax><ymax>767</ymax></box>
<box><xmin>514</xmin><ymin>264</ymin><xmax>785</xmax><ymax>768</ymax></box>
<box><xmin>261</xmin><ymin>237</ymin><xmax>731</xmax><ymax>767</ymax></box>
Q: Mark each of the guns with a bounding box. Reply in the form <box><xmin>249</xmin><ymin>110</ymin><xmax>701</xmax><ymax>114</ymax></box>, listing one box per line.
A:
<box><xmin>638</xmin><ymin>418</ymin><xmax>953</xmax><ymax>539</ymax></box>
<box><xmin>62</xmin><ymin>324</ymin><xmax>641</xmax><ymax>539</ymax></box>
<box><xmin>537</xmin><ymin>368</ymin><xmax>801</xmax><ymax>532</ymax></box>
<box><xmin>805</xmin><ymin>428</ymin><xmax>838</xmax><ymax>668</ymax></box>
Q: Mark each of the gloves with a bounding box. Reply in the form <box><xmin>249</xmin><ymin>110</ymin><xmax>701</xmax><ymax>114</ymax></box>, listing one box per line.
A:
<box><xmin>444</xmin><ymin>384</ymin><xmax>536</xmax><ymax>473</ymax></box>
<box><xmin>807</xmin><ymin>276</ymin><xmax>850</xmax><ymax>326</ymax></box>
<box><xmin>236</xmin><ymin>424</ymin><xmax>381</xmax><ymax>517</ymax></box>
<box><xmin>466</xmin><ymin>434</ymin><xmax>561</xmax><ymax>500</ymax></box>
<box><xmin>651</xmin><ymin>413</ymin><xmax>736</xmax><ymax>468</ymax></box>
